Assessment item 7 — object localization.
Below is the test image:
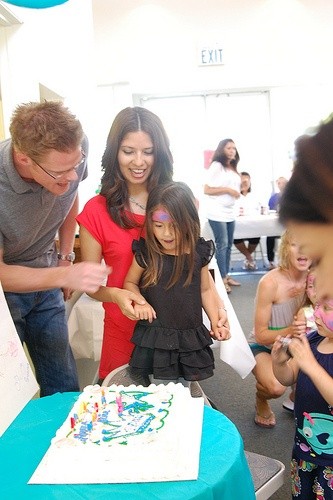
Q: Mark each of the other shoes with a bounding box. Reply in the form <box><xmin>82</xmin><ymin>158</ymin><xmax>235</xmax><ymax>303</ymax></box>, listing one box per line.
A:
<box><xmin>268</xmin><ymin>263</ymin><xmax>278</xmax><ymax>269</ymax></box>
<box><xmin>244</xmin><ymin>260</ymin><xmax>258</xmax><ymax>270</ymax></box>
<box><xmin>224</xmin><ymin>278</ymin><xmax>241</xmax><ymax>294</ymax></box>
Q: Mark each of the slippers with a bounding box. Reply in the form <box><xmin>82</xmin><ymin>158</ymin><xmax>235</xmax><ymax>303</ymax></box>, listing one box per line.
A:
<box><xmin>254</xmin><ymin>411</ymin><xmax>277</xmax><ymax>428</ymax></box>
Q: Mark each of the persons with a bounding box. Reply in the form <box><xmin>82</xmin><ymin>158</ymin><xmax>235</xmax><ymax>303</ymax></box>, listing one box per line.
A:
<box><xmin>75</xmin><ymin>106</ymin><xmax>230</xmax><ymax>389</ymax></box>
<box><xmin>277</xmin><ymin>115</ymin><xmax>333</xmax><ymax>305</ymax></box>
<box><xmin>204</xmin><ymin>138</ymin><xmax>243</xmax><ymax>292</ymax></box>
<box><xmin>0</xmin><ymin>100</ymin><xmax>114</xmax><ymax>398</ymax></box>
<box><xmin>233</xmin><ymin>172</ymin><xmax>261</xmax><ymax>269</ymax></box>
<box><xmin>266</xmin><ymin>176</ymin><xmax>288</xmax><ymax>268</ymax></box>
<box><xmin>247</xmin><ymin>228</ymin><xmax>314</xmax><ymax>425</ymax></box>
<box><xmin>122</xmin><ymin>182</ymin><xmax>232</xmax><ymax>392</ymax></box>
<box><xmin>271</xmin><ymin>295</ymin><xmax>333</xmax><ymax>500</ymax></box>
<box><xmin>297</xmin><ymin>268</ymin><xmax>319</xmax><ymax>335</ymax></box>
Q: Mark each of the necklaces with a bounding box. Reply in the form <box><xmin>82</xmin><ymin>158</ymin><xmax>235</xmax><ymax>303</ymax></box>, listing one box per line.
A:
<box><xmin>129</xmin><ymin>194</ymin><xmax>146</xmax><ymax>210</ymax></box>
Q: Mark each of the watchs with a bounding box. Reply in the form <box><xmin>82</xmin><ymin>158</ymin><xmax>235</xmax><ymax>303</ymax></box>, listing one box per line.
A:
<box><xmin>58</xmin><ymin>252</ymin><xmax>75</xmax><ymax>262</ymax></box>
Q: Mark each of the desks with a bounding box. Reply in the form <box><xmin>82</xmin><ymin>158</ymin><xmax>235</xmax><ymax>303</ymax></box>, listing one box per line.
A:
<box><xmin>234</xmin><ymin>214</ymin><xmax>283</xmax><ymax>271</ymax></box>
<box><xmin>0</xmin><ymin>392</ymin><xmax>256</xmax><ymax>499</ymax></box>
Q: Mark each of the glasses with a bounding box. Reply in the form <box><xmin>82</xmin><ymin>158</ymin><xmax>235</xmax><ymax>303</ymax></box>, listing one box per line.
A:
<box><xmin>28</xmin><ymin>151</ymin><xmax>87</xmax><ymax>181</ymax></box>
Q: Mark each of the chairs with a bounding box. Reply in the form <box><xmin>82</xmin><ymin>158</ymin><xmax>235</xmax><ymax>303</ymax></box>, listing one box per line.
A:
<box><xmin>102</xmin><ymin>363</ymin><xmax>285</xmax><ymax>500</ymax></box>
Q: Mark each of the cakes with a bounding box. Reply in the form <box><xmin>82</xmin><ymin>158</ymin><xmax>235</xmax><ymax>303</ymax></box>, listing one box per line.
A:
<box><xmin>49</xmin><ymin>382</ymin><xmax>187</xmax><ymax>467</ymax></box>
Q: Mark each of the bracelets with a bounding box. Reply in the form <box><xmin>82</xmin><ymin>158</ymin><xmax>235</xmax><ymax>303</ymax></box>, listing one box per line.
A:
<box><xmin>219</xmin><ymin>308</ymin><xmax>227</xmax><ymax>311</ymax></box>
